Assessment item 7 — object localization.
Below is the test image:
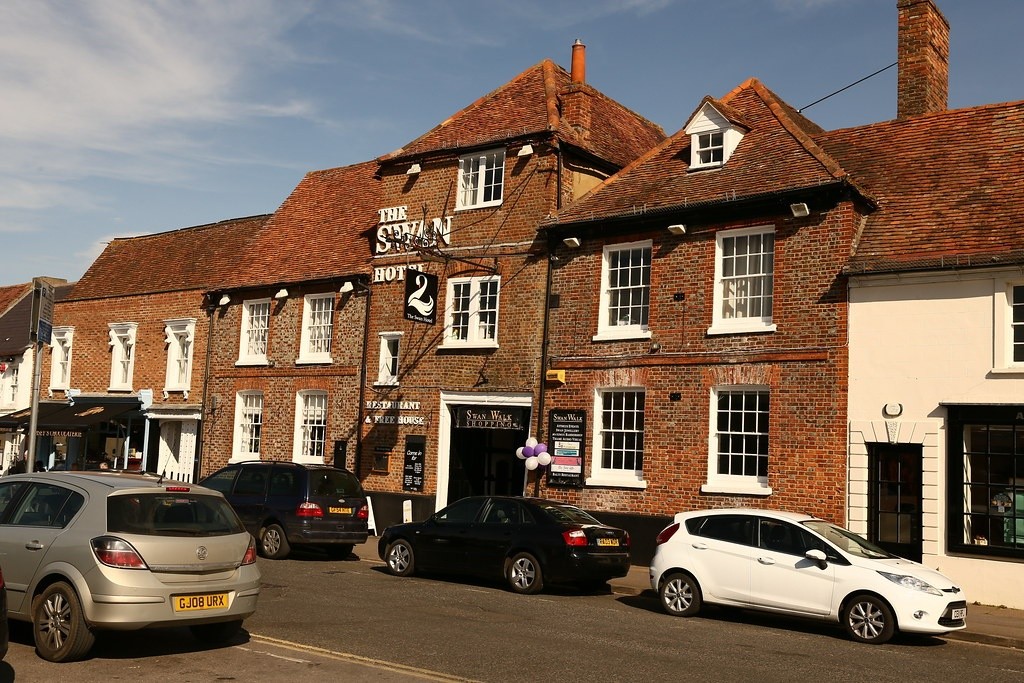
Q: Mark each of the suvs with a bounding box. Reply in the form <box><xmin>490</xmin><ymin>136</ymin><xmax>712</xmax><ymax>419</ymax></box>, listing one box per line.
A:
<box><xmin>0</xmin><ymin>441</ymin><xmax>261</xmax><ymax>664</ymax></box>
<box><xmin>199</xmin><ymin>460</ymin><xmax>368</xmax><ymax>558</ymax></box>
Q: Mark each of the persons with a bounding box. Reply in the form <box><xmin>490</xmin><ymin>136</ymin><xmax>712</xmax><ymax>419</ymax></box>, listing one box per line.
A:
<box><xmin>20</xmin><ymin>449</ymin><xmax>28</xmax><ymax>473</ymax></box>
<box><xmin>33</xmin><ymin>460</ymin><xmax>46</xmax><ymax>472</ymax></box>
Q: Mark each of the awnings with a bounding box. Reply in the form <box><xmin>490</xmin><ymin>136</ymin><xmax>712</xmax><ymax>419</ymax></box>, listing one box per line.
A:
<box><xmin>22</xmin><ymin>401</ymin><xmax>141</xmax><ymax>470</ymax></box>
<box><xmin>0</xmin><ymin>403</ymin><xmax>70</xmax><ymax>435</ymax></box>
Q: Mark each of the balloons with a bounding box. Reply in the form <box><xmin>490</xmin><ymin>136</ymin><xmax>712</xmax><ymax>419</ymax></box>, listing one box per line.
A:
<box><xmin>534</xmin><ymin>443</ymin><xmax>547</xmax><ymax>456</ymax></box>
<box><xmin>522</xmin><ymin>446</ymin><xmax>534</xmax><ymax>458</ymax></box>
<box><xmin>526</xmin><ymin>438</ymin><xmax>538</xmax><ymax>451</ymax></box>
<box><xmin>525</xmin><ymin>456</ymin><xmax>538</xmax><ymax>470</ymax></box>
<box><xmin>537</xmin><ymin>452</ymin><xmax>551</xmax><ymax>466</ymax></box>
<box><xmin>516</xmin><ymin>447</ymin><xmax>528</xmax><ymax>459</ymax></box>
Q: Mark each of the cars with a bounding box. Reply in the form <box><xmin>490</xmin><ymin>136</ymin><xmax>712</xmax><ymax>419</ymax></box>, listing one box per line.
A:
<box><xmin>377</xmin><ymin>496</ymin><xmax>632</xmax><ymax>595</ymax></box>
<box><xmin>649</xmin><ymin>507</ymin><xmax>969</xmax><ymax>645</ymax></box>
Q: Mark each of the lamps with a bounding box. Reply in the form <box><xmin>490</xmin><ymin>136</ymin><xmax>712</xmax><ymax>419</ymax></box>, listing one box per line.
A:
<box><xmin>790</xmin><ymin>204</ymin><xmax>811</xmax><ymax>217</ymax></box>
<box><xmin>517</xmin><ymin>145</ymin><xmax>537</xmax><ymax>156</ymax></box>
<box><xmin>563</xmin><ymin>238</ymin><xmax>582</xmax><ymax>247</ymax></box>
<box><xmin>407</xmin><ymin>164</ymin><xmax>421</xmax><ymax>174</ymax></box>
<box><xmin>667</xmin><ymin>224</ymin><xmax>689</xmax><ymax>235</ymax></box>
<box><xmin>219</xmin><ymin>294</ymin><xmax>231</xmax><ymax>305</ymax></box>
<box><xmin>274</xmin><ymin>289</ymin><xmax>289</xmax><ymax>299</ymax></box>
<box><xmin>340</xmin><ymin>282</ymin><xmax>354</xmax><ymax>293</ymax></box>
